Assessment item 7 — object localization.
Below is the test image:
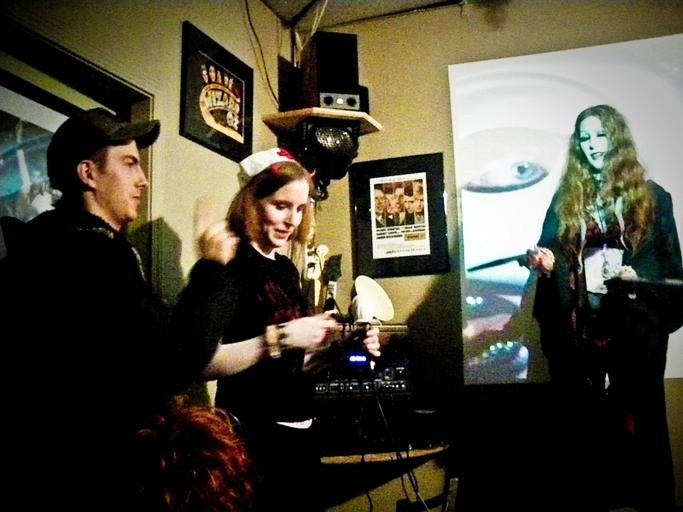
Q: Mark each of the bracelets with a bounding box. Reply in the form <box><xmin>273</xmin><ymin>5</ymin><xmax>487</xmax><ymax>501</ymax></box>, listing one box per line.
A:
<box><xmin>265</xmin><ymin>322</ymin><xmax>287</xmax><ymax>360</ymax></box>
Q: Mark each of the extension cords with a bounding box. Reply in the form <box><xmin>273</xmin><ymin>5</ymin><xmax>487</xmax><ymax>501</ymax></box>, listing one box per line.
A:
<box><xmin>303</xmin><ymin>240</ymin><xmax>317</xmax><ymax>282</ymax></box>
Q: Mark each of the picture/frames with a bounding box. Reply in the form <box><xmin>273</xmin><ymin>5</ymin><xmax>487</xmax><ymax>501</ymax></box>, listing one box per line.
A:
<box><xmin>179</xmin><ymin>20</ymin><xmax>255</xmax><ymax>163</ymax></box>
<box><xmin>347</xmin><ymin>149</ymin><xmax>451</xmax><ymax>281</ymax></box>
<box><xmin>0</xmin><ymin>14</ymin><xmax>155</xmax><ymax>292</ymax></box>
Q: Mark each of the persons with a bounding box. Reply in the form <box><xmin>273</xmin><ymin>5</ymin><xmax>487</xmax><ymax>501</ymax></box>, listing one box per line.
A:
<box><xmin>194</xmin><ymin>148</ymin><xmax>384</xmax><ymax>512</ymax></box>
<box><xmin>526</xmin><ymin>105</ymin><xmax>682</xmax><ymax>509</ymax></box>
<box><xmin>1</xmin><ymin>107</ymin><xmax>340</xmax><ymax>505</ymax></box>
<box><xmin>374</xmin><ymin>180</ymin><xmax>426</xmax><ymax>227</ymax></box>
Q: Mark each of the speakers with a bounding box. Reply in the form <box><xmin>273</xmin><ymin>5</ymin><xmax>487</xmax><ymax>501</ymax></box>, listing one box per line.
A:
<box><xmin>300</xmin><ymin>30</ymin><xmax>369</xmax><ymax>113</ymax></box>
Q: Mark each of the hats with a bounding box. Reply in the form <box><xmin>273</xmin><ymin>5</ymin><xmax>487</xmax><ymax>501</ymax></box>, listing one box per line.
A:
<box><xmin>236</xmin><ymin>147</ymin><xmax>310</xmax><ymax>191</ymax></box>
<box><xmin>46</xmin><ymin>107</ymin><xmax>162</xmax><ymax>190</ymax></box>
